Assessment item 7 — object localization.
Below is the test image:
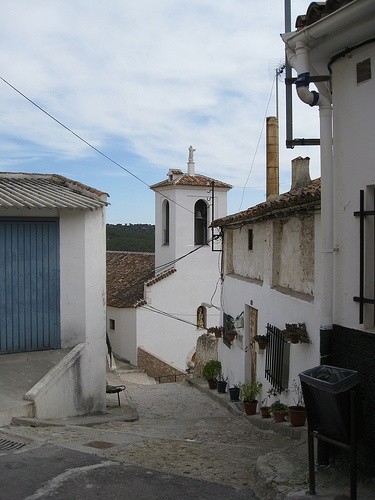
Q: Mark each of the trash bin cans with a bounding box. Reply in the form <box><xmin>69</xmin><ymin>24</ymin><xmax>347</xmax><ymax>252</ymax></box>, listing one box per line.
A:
<box><xmin>299</xmin><ymin>365</ymin><xmax>360</xmax><ymax>443</ymax></box>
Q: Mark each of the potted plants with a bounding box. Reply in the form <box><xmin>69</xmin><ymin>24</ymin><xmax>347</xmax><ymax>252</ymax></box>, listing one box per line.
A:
<box><xmin>203</xmin><ymin>359</ymin><xmax>307</xmax><ymax>427</ymax></box>
<box><xmin>282</xmin><ymin>323</ymin><xmax>312</xmax><ymax>345</ymax></box>
<box><xmin>254</xmin><ymin>334</ymin><xmax>269</xmax><ymax>349</ymax></box>
<box><xmin>225</xmin><ymin>328</ymin><xmax>238</xmax><ymax>341</ymax></box>
<box><xmin>207</xmin><ymin>326</ymin><xmax>221</xmax><ymax>338</ymax></box>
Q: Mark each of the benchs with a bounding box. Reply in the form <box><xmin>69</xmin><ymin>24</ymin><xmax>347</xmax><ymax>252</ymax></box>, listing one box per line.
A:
<box><xmin>105</xmin><ymin>384</ymin><xmax>127</xmax><ymax>406</ymax></box>
